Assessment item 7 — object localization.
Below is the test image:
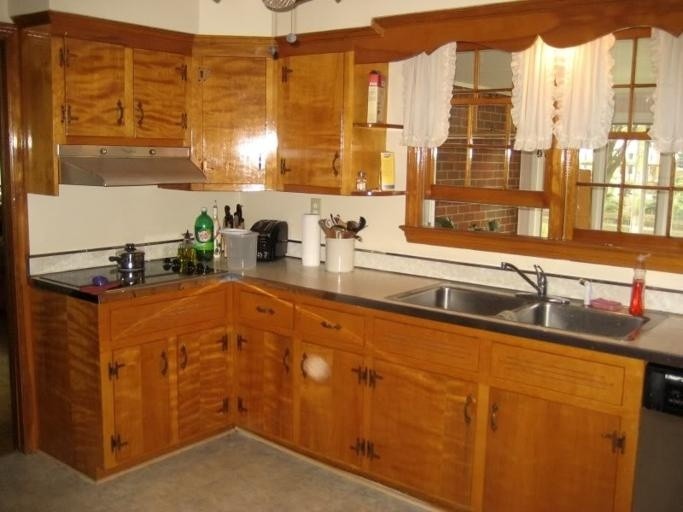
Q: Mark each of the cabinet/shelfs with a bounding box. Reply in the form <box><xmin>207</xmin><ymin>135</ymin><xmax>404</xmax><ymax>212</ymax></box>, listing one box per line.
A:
<box><xmin>10</xmin><ymin>10</ymin><xmax>191</xmax><ymax>145</ymax></box>
<box><xmin>32</xmin><ymin>277</ymin><xmax>233</xmax><ymax>482</ymax></box>
<box><xmin>230</xmin><ymin>277</ymin><xmax>368</xmax><ymax>477</ymax></box>
<box><xmin>272</xmin><ymin>27</ymin><xmax>387</xmax><ymax>192</ymax></box>
<box><xmin>369</xmin><ymin>308</ymin><xmax>647</xmax><ymax>512</ymax></box>
<box><xmin>159</xmin><ymin>33</ymin><xmax>272</xmax><ymax>192</ymax></box>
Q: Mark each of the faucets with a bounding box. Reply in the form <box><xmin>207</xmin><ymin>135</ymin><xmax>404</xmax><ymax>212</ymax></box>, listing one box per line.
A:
<box><xmin>501</xmin><ymin>261</ymin><xmax>570</xmax><ymax>305</ymax></box>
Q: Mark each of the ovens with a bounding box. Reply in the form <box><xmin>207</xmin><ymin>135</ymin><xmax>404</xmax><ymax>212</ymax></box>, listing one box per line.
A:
<box><xmin>632</xmin><ymin>364</ymin><xmax>681</xmax><ymax>512</ymax></box>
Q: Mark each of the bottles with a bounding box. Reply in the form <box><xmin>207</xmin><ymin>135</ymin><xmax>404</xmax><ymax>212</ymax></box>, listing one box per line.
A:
<box><xmin>194</xmin><ymin>207</ymin><xmax>214</xmax><ymax>259</ymax></box>
<box><xmin>629</xmin><ymin>254</ymin><xmax>650</xmax><ymax>317</ymax></box>
<box><xmin>213</xmin><ymin>201</ymin><xmax>222</xmax><ymax>259</ymax></box>
<box><xmin>177</xmin><ymin>229</ymin><xmax>195</xmax><ymax>260</ymax></box>
<box><xmin>356</xmin><ymin>171</ymin><xmax>368</xmax><ymax>191</ymax></box>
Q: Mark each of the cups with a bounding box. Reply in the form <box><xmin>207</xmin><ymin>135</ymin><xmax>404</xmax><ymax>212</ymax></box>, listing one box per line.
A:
<box><xmin>325</xmin><ymin>239</ymin><xmax>354</xmax><ymax>273</ymax></box>
<box><xmin>221</xmin><ymin>227</ymin><xmax>259</xmax><ymax>270</ymax></box>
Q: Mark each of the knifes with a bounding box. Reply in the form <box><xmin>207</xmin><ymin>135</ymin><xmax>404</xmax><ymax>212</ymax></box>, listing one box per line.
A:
<box><xmin>224</xmin><ymin>203</ymin><xmax>244</xmax><ymax>228</ymax></box>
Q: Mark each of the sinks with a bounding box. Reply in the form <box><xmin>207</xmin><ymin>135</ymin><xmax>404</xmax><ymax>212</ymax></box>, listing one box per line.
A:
<box><xmin>516</xmin><ymin>301</ymin><xmax>651</xmax><ymax>341</ymax></box>
<box><xmin>384</xmin><ymin>280</ymin><xmax>526</xmax><ymax>319</ymax></box>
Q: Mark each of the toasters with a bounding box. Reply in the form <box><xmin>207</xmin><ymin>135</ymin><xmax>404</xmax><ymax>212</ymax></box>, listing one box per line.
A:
<box><xmin>250</xmin><ymin>219</ymin><xmax>288</xmax><ymax>261</ymax></box>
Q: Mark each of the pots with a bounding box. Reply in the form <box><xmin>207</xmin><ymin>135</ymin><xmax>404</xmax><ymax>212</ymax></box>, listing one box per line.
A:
<box><xmin>108</xmin><ymin>242</ymin><xmax>144</xmax><ymax>272</ymax></box>
<box><xmin>116</xmin><ymin>271</ymin><xmax>144</xmax><ymax>284</ymax></box>
